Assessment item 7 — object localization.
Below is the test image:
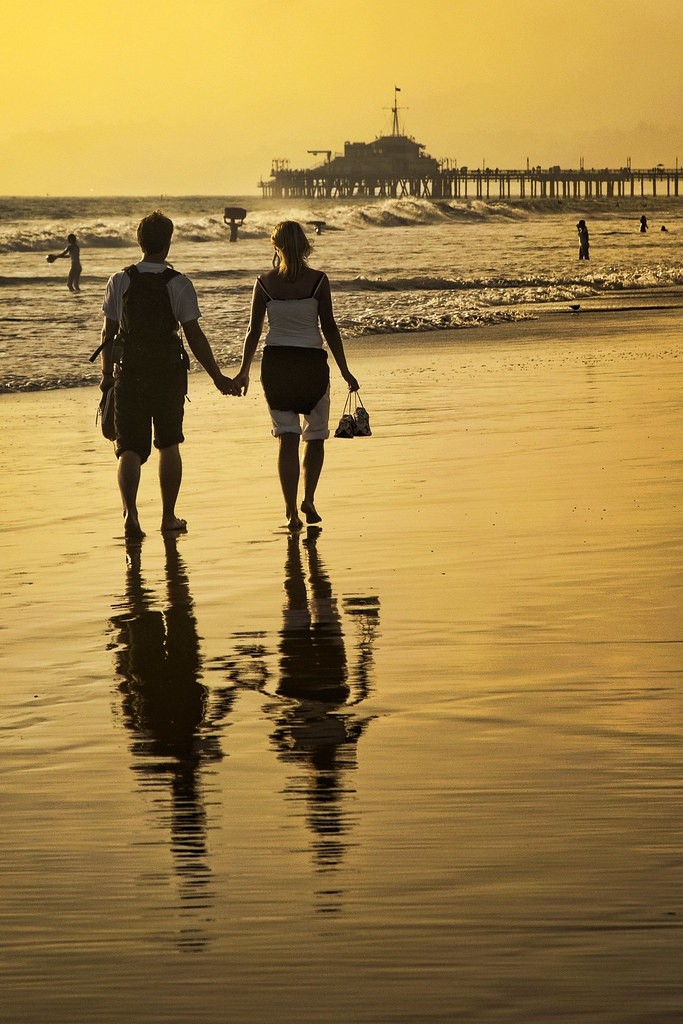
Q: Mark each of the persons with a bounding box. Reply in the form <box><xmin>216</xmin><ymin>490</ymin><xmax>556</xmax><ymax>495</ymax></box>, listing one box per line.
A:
<box><xmin>99</xmin><ymin>210</ymin><xmax>232</xmax><ymax>537</ymax></box>
<box><xmin>232</xmin><ymin>220</ymin><xmax>360</xmax><ymax>527</ymax></box>
<box><xmin>661</xmin><ymin>226</ymin><xmax>667</xmax><ymax>231</ymax></box>
<box><xmin>223</xmin><ymin>217</ymin><xmax>243</xmax><ymax>242</ymax></box>
<box><xmin>275</xmin><ymin>526</ymin><xmax>369</xmax><ymax>838</ymax></box>
<box><xmin>113</xmin><ymin>541</ymin><xmax>237</xmax><ymax>860</ymax></box>
<box><xmin>640</xmin><ymin>215</ymin><xmax>648</xmax><ymax>232</ymax></box>
<box><xmin>46</xmin><ymin>234</ymin><xmax>82</xmax><ymax>291</ymax></box>
<box><xmin>576</xmin><ymin>220</ymin><xmax>589</xmax><ymax>260</ymax></box>
<box><xmin>314</xmin><ymin>225</ymin><xmax>325</xmax><ymax>235</ymax></box>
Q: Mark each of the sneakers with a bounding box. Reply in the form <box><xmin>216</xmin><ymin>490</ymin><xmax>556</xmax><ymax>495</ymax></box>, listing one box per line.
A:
<box><xmin>352</xmin><ymin>390</ymin><xmax>372</xmax><ymax>436</ymax></box>
<box><xmin>96</xmin><ymin>384</ymin><xmax>116</xmax><ymax>441</ymax></box>
<box><xmin>333</xmin><ymin>391</ymin><xmax>354</xmax><ymax>438</ymax></box>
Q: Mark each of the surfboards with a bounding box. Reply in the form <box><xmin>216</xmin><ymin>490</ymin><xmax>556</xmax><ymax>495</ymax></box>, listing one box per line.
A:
<box><xmin>224</xmin><ymin>207</ymin><xmax>246</xmax><ymax>219</ymax></box>
<box><xmin>306</xmin><ymin>221</ymin><xmax>327</xmax><ymax>225</ymax></box>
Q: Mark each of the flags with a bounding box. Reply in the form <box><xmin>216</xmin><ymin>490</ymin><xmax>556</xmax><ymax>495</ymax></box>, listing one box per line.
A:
<box><xmin>396</xmin><ymin>88</ymin><xmax>401</xmax><ymax>91</ymax></box>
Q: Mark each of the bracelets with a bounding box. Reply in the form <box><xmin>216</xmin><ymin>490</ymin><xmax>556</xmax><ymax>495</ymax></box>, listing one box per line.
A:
<box><xmin>102</xmin><ymin>369</ymin><xmax>114</xmax><ymax>374</ymax></box>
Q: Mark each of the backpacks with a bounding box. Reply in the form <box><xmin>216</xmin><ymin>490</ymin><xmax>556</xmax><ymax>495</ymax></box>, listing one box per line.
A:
<box><xmin>110</xmin><ymin>267</ymin><xmax>183</xmax><ymax>373</ymax></box>
